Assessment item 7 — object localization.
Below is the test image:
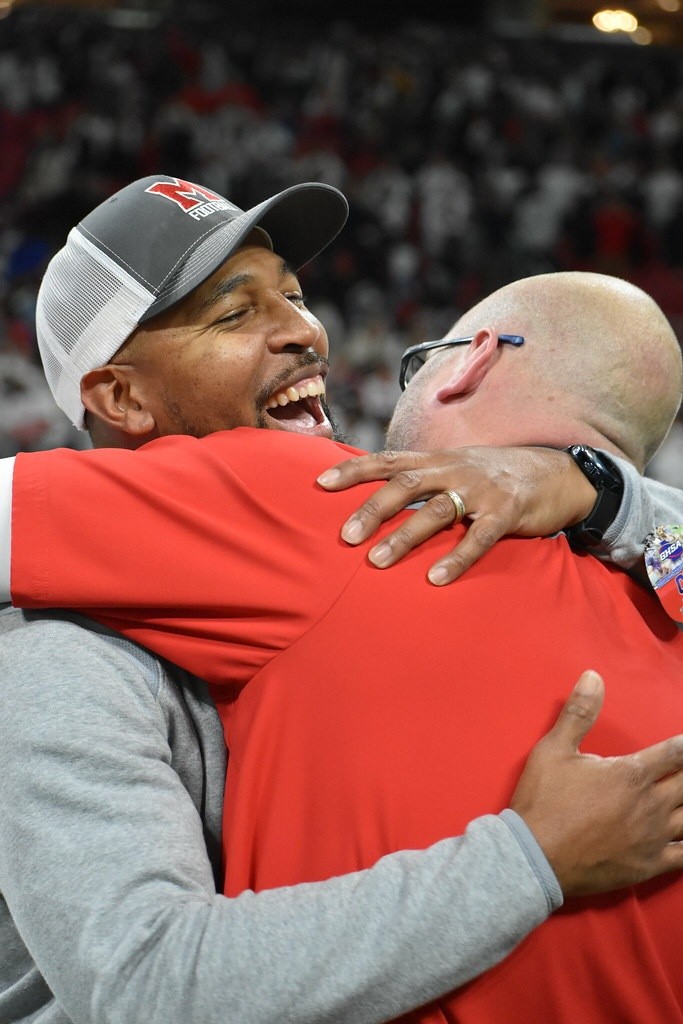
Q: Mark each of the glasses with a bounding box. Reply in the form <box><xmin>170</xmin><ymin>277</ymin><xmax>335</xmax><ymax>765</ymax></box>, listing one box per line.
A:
<box><xmin>399</xmin><ymin>333</ymin><xmax>524</xmax><ymax>393</ymax></box>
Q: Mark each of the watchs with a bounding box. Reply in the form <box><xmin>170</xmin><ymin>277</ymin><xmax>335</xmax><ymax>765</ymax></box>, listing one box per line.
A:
<box><xmin>562</xmin><ymin>443</ymin><xmax>624</xmax><ymax>555</ymax></box>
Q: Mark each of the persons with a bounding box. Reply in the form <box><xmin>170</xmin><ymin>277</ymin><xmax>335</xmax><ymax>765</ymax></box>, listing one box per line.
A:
<box><xmin>0</xmin><ymin>174</ymin><xmax>683</xmax><ymax>1024</ymax></box>
<box><xmin>0</xmin><ymin>271</ymin><xmax>683</xmax><ymax>1023</ymax></box>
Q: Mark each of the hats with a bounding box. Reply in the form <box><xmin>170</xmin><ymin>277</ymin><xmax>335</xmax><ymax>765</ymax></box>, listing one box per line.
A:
<box><xmin>34</xmin><ymin>173</ymin><xmax>349</xmax><ymax>434</ymax></box>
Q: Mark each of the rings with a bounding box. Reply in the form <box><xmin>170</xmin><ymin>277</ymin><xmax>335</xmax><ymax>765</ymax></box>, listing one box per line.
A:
<box><xmin>444</xmin><ymin>490</ymin><xmax>465</xmax><ymax>525</ymax></box>
